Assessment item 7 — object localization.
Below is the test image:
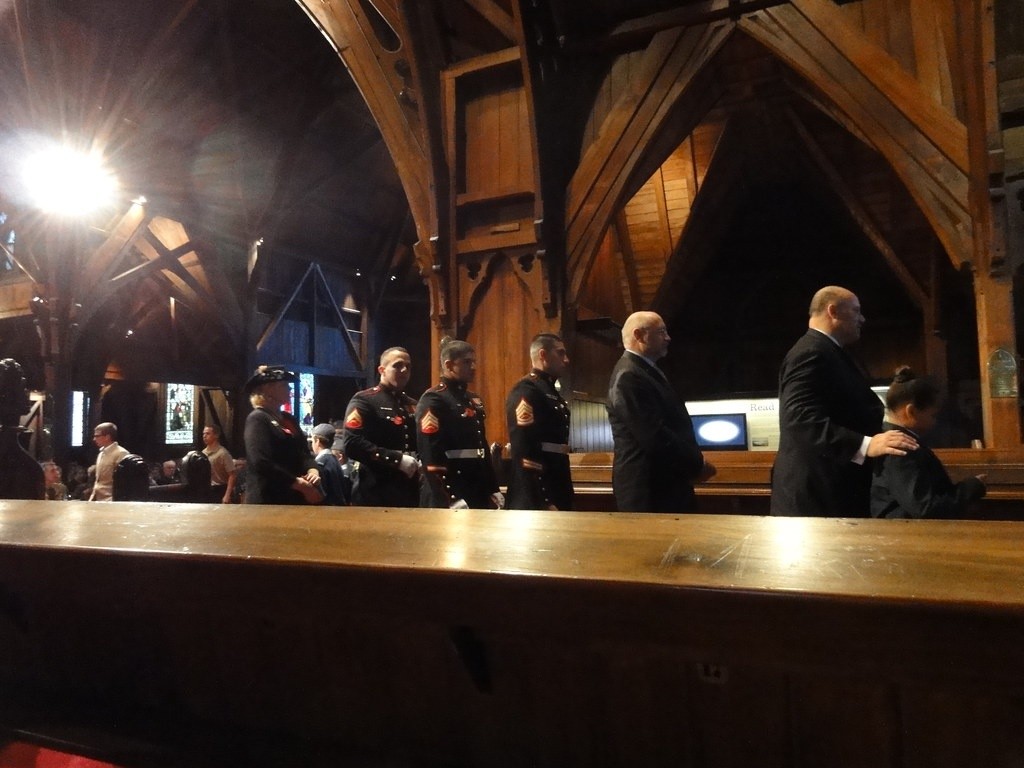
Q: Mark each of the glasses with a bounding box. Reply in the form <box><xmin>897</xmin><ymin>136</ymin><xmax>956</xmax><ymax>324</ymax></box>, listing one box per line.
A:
<box><xmin>92</xmin><ymin>433</ymin><xmax>107</xmax><ymax>438</ymax></box>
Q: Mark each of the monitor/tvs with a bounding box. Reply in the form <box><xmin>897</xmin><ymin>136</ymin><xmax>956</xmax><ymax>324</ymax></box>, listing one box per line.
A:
<box><xmin>691</xmin><ymin>413</ymin><xmax>748</xmax><ymax>451</ymax></box>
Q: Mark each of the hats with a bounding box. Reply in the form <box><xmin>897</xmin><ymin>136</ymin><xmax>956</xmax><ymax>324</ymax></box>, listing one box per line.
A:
<box><xmin>311</xmin><ymin>423</ymin><xmax>335</xmax><ymax>441</ymax></box>
<box><xmin>331</xmin><ymin>438</ymin><xmax>344</xmax><ymax>452</ymax></box>
<box><xmin>240</xmin><ymin>365</ymin><xmax>300</xmax><ymax>394</ymax></box>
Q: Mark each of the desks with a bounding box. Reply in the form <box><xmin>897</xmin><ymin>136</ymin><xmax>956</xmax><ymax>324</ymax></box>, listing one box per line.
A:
<box><xmin>0</xmin><ymin>499</ymin><xmax>1024</xmax><ymax>767</ymax></box>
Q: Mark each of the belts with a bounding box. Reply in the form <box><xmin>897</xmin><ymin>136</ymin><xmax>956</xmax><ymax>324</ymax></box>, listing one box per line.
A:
<box><xmin>542</xmin><ymin>441</ymin><xmax>571</xmax><ymax>454</ymax></box>
<box><xmin>395</xmin><ymin>449</ymin><xmax>419</xmax><ymax>462</ymax></box>
<box><xmin>445</xmin><ymin>448</ymin><xmax>488</xmax><ymax>459</ymax></box>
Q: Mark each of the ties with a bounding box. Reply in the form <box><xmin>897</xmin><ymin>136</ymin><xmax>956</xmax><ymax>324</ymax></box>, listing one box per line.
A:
<box><xmin>653</xmin><ymin>364</ymin><xmax>669</xmax><ymax>385</ymax></box>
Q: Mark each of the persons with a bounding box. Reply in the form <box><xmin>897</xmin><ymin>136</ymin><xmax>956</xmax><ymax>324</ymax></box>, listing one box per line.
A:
<box><xmin>413</xmin><ymin>340</ymin><xmax>506</xmax><ymax>510</ymax></box>
<box><xmin>244</xmin><ymin>364</ymin><xmax>323</xmax><ymax>506</ymax></box>
<box><xmin>605</xmin><ymin>310</ymin><xmax>717</xmax><ymax>514</ymax></box>
<box><xmin>342</xmin><ymin>346</ymin><xmax>420</xmax><ymax>508</ymax></box>
<box><xmin>869</xmin><ymin>365</ymin><xmax>988</xmax><ymax>519</ymax></box>
<box><xmin>770</xmin><ymin>285</ymin><xmax>920</xmax><ymax>517</ymax></box>
<box><xmin>39</xmin><ymin>421</ymin><xmax>248</xmax><ymax>504</ymax></box>
<box><xmin>506</xmin><ymin>333</ymin><xmax>574</xmax><ymax>511</ymax></box>
<box><xmin>311</xmin><ymin>423</ymin><xmax>355</xmax><ymax>507</ymax></box>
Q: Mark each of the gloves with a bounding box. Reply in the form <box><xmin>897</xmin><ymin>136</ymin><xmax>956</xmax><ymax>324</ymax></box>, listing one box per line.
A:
<box><xmin>449</xmin><ymin>499</ymin><xmax>469</xmax><ymax>510</ymax></box>
<box><xmin>492</xmin><ymin>492</ymin><xmax>505</xmax><ymax>509</ymax></box>
<box><xmin>399</xmin><ymin>454</ymin><xmax>417</xmax><ymax>478</ymax></box>
